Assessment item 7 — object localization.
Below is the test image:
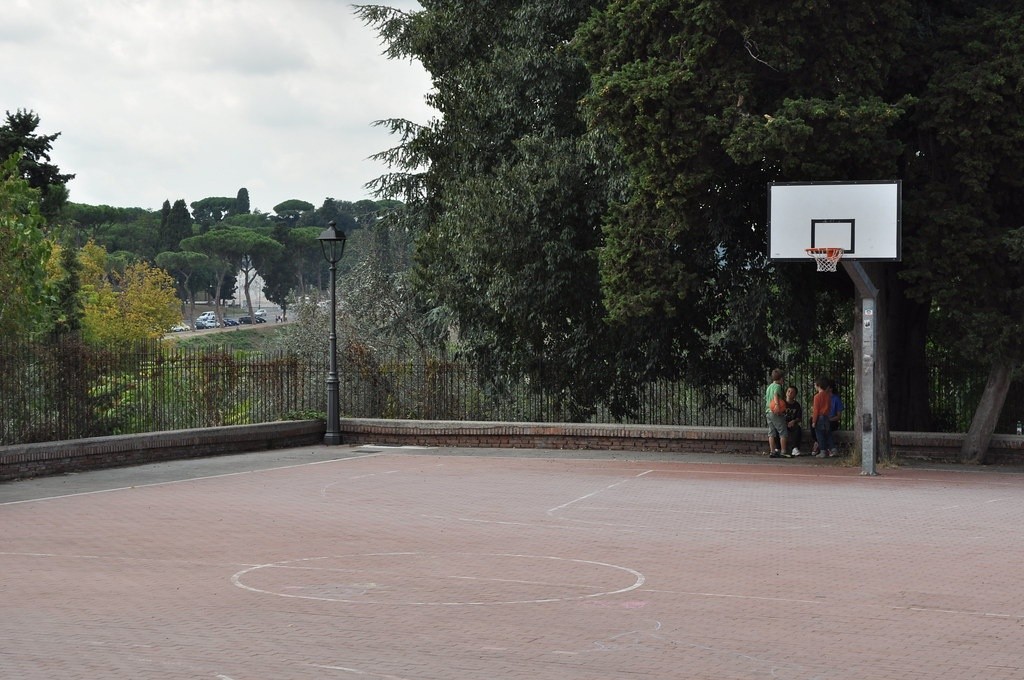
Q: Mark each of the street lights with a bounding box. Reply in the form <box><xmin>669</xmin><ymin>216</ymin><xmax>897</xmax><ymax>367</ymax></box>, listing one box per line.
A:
<box><xmin>316</xmin><ymin>219</ymin><xmax>348</xmax><ymax>445</ymax></box>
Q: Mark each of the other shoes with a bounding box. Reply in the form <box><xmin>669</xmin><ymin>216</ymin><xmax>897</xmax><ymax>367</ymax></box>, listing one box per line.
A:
<box><xmin>769</xmin><ymin>452</ymin><xmax>781</xmax><ymax>458</ymax></box>
<box><xmin>812</xmin><ymin>445</ymin><xmax>838</xmax><ymax>458</ymax></box>
<box><xmin>779</xmin><ymin>451</ymin><xmax>792</xmax><ymax>458</ymax></box>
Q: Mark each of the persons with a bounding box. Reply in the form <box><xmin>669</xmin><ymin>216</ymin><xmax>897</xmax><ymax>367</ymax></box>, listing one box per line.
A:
<box><xmin>773</xmin><ymin>385</ymin><xmax>804</xmax><ymax>457</ymax></box>
<box><xmin>809</xmin><ymin>379</ymin><xmax>845</xmax><ymax>457</ymax></box>
<box><xmin>231</xmin><ymin>300</ymin><xmax>235</xmax><ymax>310</ymax></box>
<box><xmin>763</xmin><ymin>367</ymin><xmax>796</xmax><ymax>459</ymax></box>
<box><xmin>812</xmin><ymin>376</ymin><xmax>840</xmax><ymax>458</ymax></box>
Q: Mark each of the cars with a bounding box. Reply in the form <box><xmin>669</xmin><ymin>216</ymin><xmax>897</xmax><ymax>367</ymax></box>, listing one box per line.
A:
<box><xmin>162</xmin><ymin>300</ymin><xmax>288</xmax><ymax>332</ymax></box>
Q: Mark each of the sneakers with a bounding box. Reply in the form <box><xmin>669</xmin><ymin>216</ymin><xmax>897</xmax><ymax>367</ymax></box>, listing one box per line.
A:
<box><xmin>792</xmin><ymin>447</ymin><xmax>799</xmax><ymax>456</ymax></box>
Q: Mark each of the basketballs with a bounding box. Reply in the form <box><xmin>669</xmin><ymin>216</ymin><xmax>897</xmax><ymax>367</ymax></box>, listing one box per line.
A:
<box><xmin>769</xmin><ymin>399</ymin><xmax>786</xmax><ymax>414</ymax></box>
